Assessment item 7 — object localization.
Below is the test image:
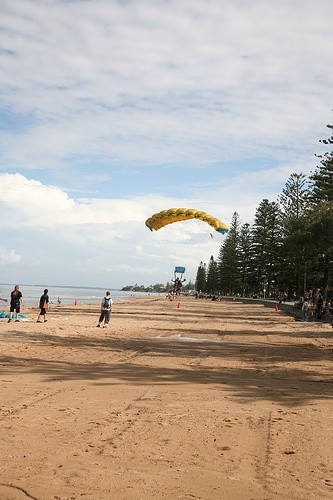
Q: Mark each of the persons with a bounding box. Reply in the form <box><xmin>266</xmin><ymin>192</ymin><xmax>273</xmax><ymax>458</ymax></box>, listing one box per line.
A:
<box><xmin>37</xmin><ymin>289</ymin><xmax>50</xmax><ymax>323</ymax></box>
<box><xmin>96</xmin><ymin>290</ymin><xmax>112</xmax><ymax>329</ymax></box>
<box><xmin>171</xmin><ymin>278</ymin><xmax>186</xmax><ymax>296</ymax></box>
<box><xmin>277</xmin><ymin>288</ymin><xmax>332</xmax><ymax>322</ymax></box>
<box><xmin>253</xmin><ymin>293</ymin><xmax>257</xmax><ymax>299</ymax></box>
<box><xmin>7</xmin><ymin>285</ymin><xmax>24</xmax><ymax>323</ymax></box>
<box><xmin>195</xmin><ymin>291</ymin><xmax>223</xmax><ymax>302</ymax></box>
<box><xmin>166</xmin><ymin>293</ymin><xmax>178</xmax><ymax>301</ymax></box>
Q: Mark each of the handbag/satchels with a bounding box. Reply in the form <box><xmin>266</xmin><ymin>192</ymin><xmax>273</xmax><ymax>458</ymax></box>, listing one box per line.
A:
<box><xmin>43</xmin><ymin>299</ymin><xmax>47</xmax><ymax>308</ymax></box>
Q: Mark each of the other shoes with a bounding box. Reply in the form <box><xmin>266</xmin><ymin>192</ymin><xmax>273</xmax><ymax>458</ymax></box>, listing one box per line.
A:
<box><xmin>44</xmin><ymin>320</ymin><xmax>47</xmax><ymax>322</ymax></box>
<box><xmin>97</xmin><ymin>325</ymin><xmax>100</xmax><ymax>327</ymax></box>
<box><xmin>15</xmin><ymin>320</ymin><xmax>20</xmax><ymax>322</ymax></box>
<box><xmin>37</xmin><ymin>321</ymin><xmax>42</xmax><ymax>323</ymax></box>
<box><xmin>8</xmin><ymin>319</ymin><xmax>11</xmax><ymax>323</ymax></box>
<box><xmin>103</xmin><ymin>326</ymin><xmax>106</xmax><ymax>328</ymax></box>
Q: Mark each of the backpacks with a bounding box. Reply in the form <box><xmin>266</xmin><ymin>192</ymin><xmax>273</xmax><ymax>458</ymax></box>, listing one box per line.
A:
<box><xmin>103</xmin><ymin>297</ymin><xmax>111</xmax><ymax>308</ymax></box>
